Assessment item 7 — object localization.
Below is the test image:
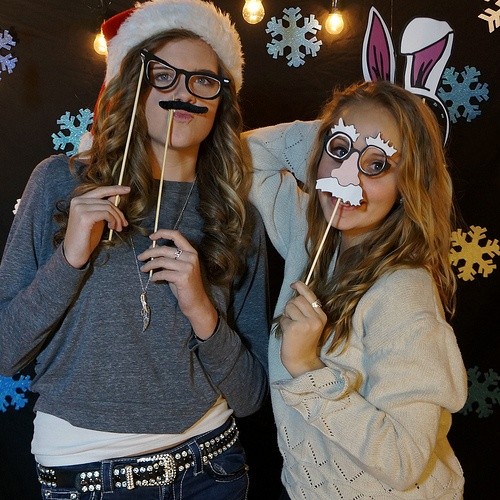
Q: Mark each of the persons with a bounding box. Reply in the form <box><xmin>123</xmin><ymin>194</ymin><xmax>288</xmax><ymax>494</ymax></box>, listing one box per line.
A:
<box><xmin>0</xmin><ymin>0</ymin><xmax>269</xmax><ymax>500</ymax></box>
<box><xmin>236</xmin><ymin>81</ymin><xmax>467</xmax><ymax>500</ymax></box>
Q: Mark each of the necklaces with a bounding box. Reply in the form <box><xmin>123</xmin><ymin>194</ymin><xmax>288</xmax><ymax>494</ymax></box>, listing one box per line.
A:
<box><xmin>128</xmin><ymin>172</ymin><xmax>195</xmax><ymax>332</ymax></box>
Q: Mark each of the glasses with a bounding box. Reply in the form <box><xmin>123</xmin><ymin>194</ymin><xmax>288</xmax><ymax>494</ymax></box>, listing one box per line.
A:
<box><xmin>324</xmin><ymin>131</ymin><xmax>391</xmax><ymax>177</ymax></box>
<box><xmin>141</xmin><ymin>48</ymin><xmax>231</xmax><ymax>100</ymax></box>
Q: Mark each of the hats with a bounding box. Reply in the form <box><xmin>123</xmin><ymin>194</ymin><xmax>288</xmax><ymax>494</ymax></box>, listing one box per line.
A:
<box><xmin>101</xmin><ymin>0</ymin><xmax>244</xmax><ymax>93</ymax></box>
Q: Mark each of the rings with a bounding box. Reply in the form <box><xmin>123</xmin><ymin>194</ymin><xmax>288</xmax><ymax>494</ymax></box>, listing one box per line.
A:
<box><xmin>174</xmin><ymin>248</ymin><xmax>183</xmax><ymax>260</ymax></box>
<box><xmin>312</xmin><ymin>298</ymin><xmax>323</xmax><ymax>309</ymax></box>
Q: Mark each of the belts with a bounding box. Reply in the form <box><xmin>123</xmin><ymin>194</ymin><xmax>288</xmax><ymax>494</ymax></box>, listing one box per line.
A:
<box><xmin>37</xmin><ymin>416</ymin><xmax>239</xmax><ymax>494</ymax></box>
